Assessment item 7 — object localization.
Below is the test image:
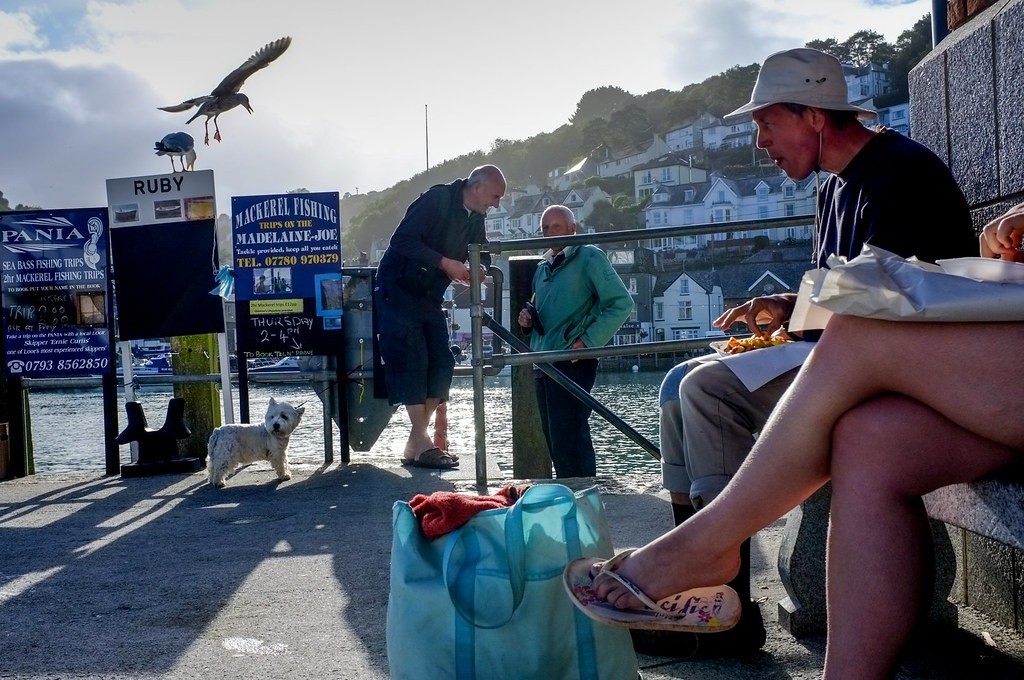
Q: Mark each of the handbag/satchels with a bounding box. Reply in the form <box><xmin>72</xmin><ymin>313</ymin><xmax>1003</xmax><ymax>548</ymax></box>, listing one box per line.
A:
<box><xmin>386</xmin><ymin>486</ymin><xmax>637</xmax><ymax>680</ymax></box>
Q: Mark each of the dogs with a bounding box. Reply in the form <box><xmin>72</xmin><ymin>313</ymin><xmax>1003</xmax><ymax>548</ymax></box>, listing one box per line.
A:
<box><xmin>206</xmin><ymin>396</ymin><xmax>305</xmax><ymax>488</ymax></box>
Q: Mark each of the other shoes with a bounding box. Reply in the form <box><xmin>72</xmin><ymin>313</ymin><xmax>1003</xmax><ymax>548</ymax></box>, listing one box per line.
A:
<box><xmin>632</xmin><ymin>594</ymin><xmax>766</xmax><ymax>659</ymax></box>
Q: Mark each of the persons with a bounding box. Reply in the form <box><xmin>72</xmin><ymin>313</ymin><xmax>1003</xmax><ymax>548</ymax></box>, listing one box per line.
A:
<box><xmin>556</xmin><ymin>196</ymin><xmax>1021</xmax><ymax>680</ymax></box>
<box><xmin>516</xmin><ymin>203</ymin><xmax>636</xmax><ymax>483</ymax></box>
<box><xmin>371</xmin><ymin>165</ymin><xmax>508</xmax><ymax>467</ymax></box>
<box><xmin>627</xmin><ymin>45</ymin><xmax>982</xmax><ymax>663</ymax></box>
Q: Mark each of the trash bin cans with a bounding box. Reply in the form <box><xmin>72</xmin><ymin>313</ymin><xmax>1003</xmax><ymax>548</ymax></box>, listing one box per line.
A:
<box><xmin>0</xmin><ymin>421</ymin><xmax>10</xmax><ymax>478</ymax></box>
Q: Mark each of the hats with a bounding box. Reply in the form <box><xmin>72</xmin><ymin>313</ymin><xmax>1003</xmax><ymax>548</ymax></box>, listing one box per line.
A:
<box><xmin>722</xmin><ymin>47</ymin><xmax>878</xmax><ymax>120</ymax></box>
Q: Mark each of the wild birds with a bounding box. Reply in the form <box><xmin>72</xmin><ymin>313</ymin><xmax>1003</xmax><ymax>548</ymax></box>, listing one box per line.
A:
<box><xmin>155</xmin><ymin>35</ymin><xmax>291</xmax><ymax>146</ymax></box>
<box><xmin>154</xmin><ymin>131</ymin><xmax>197</xmax><ymax>174</ymax></box>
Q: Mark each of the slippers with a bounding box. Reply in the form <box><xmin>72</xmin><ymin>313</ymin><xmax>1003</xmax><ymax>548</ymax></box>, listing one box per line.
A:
<box><xmin>563</xmin><ymin>548</ymin><xmax>741</xmax><ymax>633</ymax></box>
<box><xmin>401</xmin><ymin>444</ymin><xmax>460</xmax><ymax>468</ymax></box>
